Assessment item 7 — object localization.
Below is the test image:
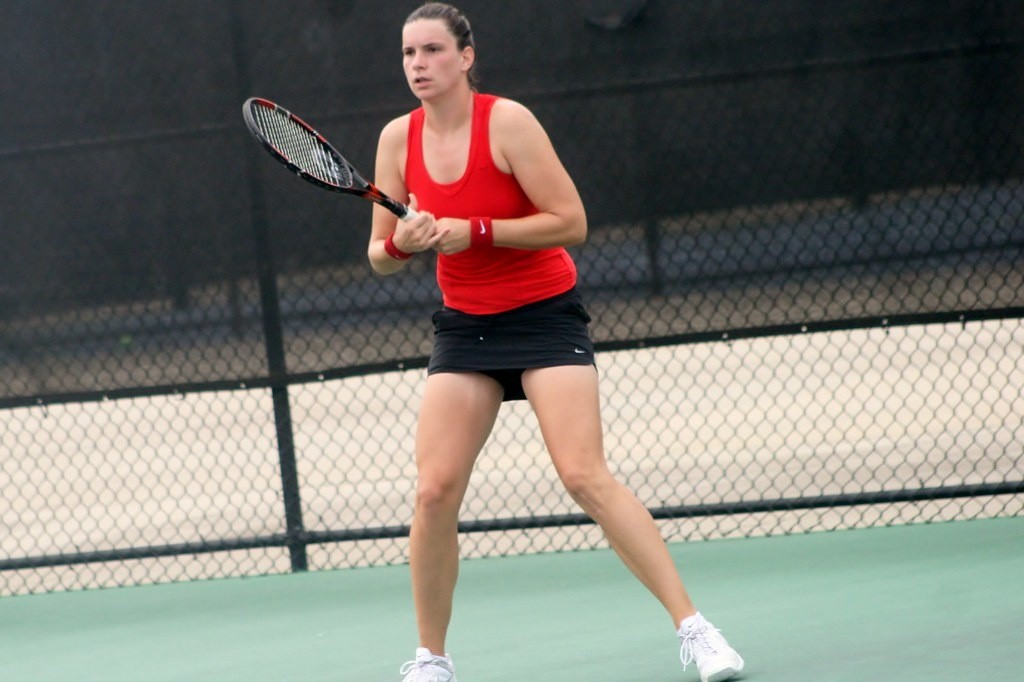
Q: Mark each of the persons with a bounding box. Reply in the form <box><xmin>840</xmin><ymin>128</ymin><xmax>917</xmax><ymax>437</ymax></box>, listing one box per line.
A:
<box><xmin>367</xmin><ymin>4</ymin><xmax>747</xmax><ymax>682</ymax></box>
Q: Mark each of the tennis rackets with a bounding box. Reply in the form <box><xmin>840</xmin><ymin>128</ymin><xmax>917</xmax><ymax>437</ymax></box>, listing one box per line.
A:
<box><xmin>240</xmin><ymin>94</ymin><xmax>420</xmax><ymax>221</ymax></box>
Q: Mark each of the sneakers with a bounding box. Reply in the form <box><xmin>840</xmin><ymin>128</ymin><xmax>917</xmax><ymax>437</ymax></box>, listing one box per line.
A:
<box><xmin>400</xmin><ymin>647</ymin><xmax>456</xmax><ymax>682</ymax></box>
<box><xmin>675</xmin><ymin>611</ymin><xmax>744</xmax><ymax>682</ymax></box>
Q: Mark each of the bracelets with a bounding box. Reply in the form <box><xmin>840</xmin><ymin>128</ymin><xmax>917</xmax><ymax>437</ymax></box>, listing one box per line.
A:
<box><xmin>384</xmin><ymin>232</ymin><xmax>414</xmax><ymax>261</ymax></box>
<box><xmin>468</xmin><ymin>216</ymin><xmax>494</xmax><ymax>248</ymax></box>
